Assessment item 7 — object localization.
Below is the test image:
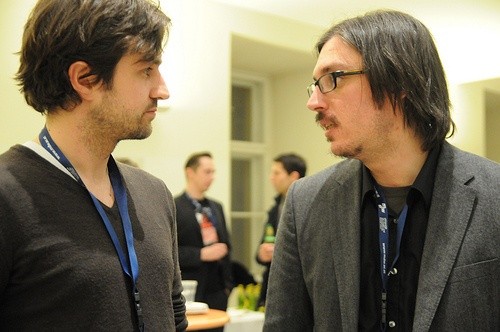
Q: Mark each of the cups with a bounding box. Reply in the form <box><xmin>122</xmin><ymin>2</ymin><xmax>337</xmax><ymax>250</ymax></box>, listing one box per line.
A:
<box><xmin>181</xmin><ymin>279</ymin><xmax>198</xmax><ymax>301</ymax></box>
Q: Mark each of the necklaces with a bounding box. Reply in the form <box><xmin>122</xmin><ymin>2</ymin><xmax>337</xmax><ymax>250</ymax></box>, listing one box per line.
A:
<box><xmin>373</xmin><ymin>185</ymin><xmax>408</xmax><ymax>332</ymax></box>
<box><xmin>39</xmin><ymin>125</ymin><xmax>144</xmax><ymax>332</ymax></box>
<box><xmin>188</xmin><ymin>194</ymin><xmax>229</xmax><ymax>242</ymax></box>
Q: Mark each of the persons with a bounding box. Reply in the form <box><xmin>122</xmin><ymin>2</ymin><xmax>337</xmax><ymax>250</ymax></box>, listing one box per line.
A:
<box><xmin>261</xmin><ymin>8</ymin><xmax>500</xmax><ymax>331</ymax></box>
<box><xmin>255</xmin><ymin>153</ymin><xmax>306</xmax><ymax>311</ymax></box>
<box><xmin>0</xmin><ymin>1</ymin><xmax>188</xmax><ymax>332</ymax></box>
<box><xmin>173</xmin><ymin>154</ymin><xmax>231</xmax><ymax>332</ymax></box>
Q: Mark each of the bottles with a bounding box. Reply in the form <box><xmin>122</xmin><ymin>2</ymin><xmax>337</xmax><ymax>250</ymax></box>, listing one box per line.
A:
<box><xmin>264</xmin><ymin>223</ymin><xmax>275</xmax><ymax>243</ymax></box>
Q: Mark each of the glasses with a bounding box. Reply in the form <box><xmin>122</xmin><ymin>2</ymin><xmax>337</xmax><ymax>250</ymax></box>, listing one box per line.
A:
<box><xmin>307</xmin><ymin>70</ymin><xmax>367</xmax><ymax>98</ymax></box>
